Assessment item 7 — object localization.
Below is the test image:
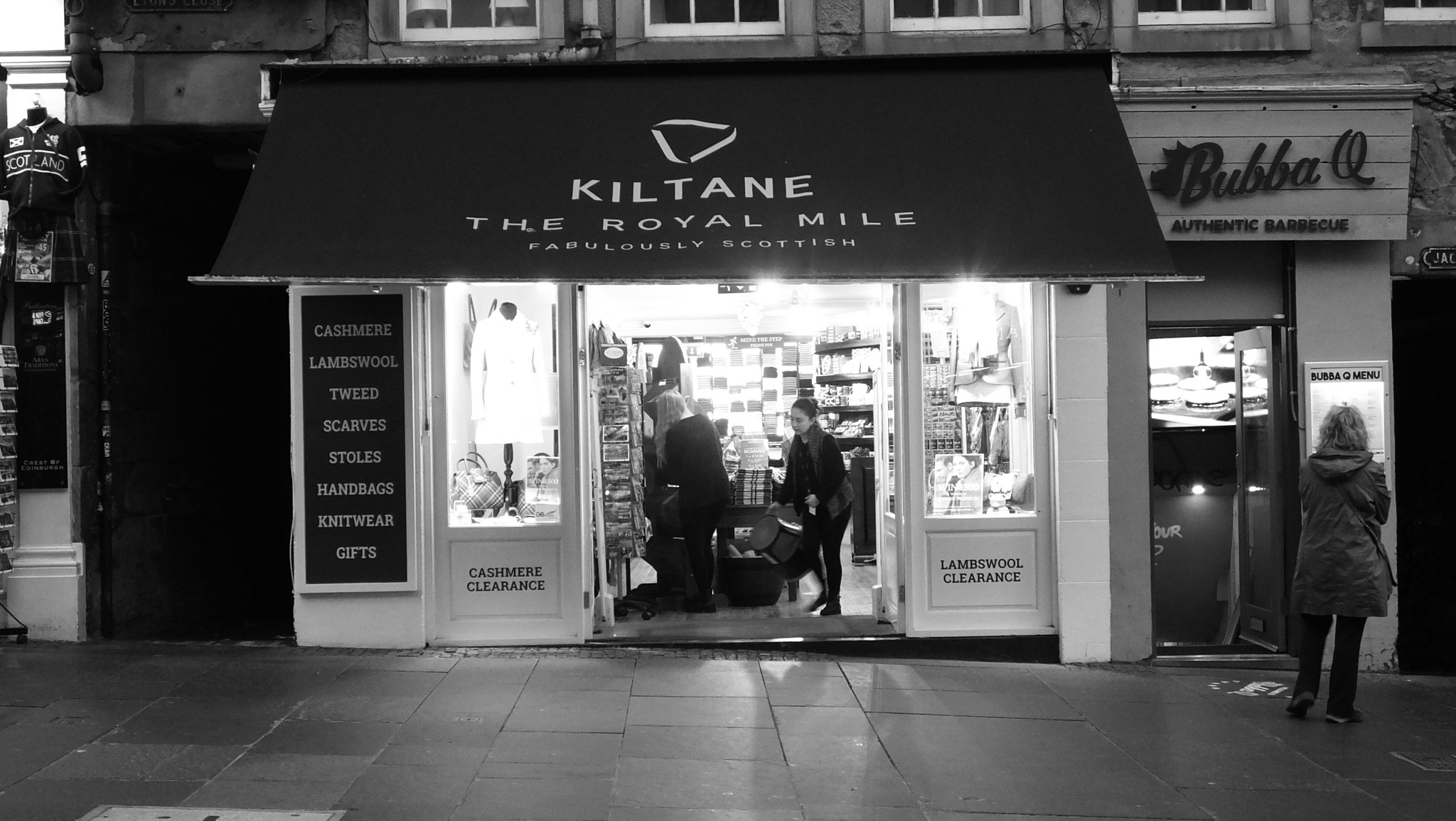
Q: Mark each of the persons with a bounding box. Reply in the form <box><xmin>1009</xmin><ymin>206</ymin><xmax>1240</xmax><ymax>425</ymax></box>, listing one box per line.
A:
<box><xmin>654</xmin><ymin>391</ymin><xmax>732</xmax><ymax>613</ymax></box>
<box><xmin>1285</xmin><ymin>404</ymin><xmax>1400</xmax><ymax>721</ymax></box>
<box><xmin>0</xmin><ymin>107</ymin><xmax>91</xmax><ymax>238</ymax></box>
<box><xmin>936</xmin><ymin>454</ymin><xmax>982</xmax><ymax>516</ymax></box>
<box><xmin>947</xmin><ymin>288</ymin><xmax>1025</xmax><ymax>469</ymax></box>
<box><xmin>765</xmin><ymin>398</ymin><xmax>855</xmax><ymax>615</ymax></box>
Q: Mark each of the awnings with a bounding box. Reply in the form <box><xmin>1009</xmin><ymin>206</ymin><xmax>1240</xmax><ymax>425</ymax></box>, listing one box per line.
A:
<box><xmin>186</xmin><ymin>47</ymin><xmax>1201</xmax><ymax>281</ymax></box>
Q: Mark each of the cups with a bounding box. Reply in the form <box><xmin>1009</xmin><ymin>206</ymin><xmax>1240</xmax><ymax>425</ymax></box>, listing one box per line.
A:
<box><xmin>829</xmin><ymin>383</ymin><xmax>873</xmax><ymax>406</ymax></box>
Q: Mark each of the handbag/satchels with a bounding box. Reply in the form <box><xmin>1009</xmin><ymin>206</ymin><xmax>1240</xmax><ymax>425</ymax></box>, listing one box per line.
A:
<box><xmin>451</xmin><ymin>452</ymin><xmax>505</xmax><ymax>508</ymax></box>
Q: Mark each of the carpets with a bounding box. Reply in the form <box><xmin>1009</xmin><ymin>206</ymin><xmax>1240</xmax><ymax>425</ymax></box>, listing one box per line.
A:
<box><xmin>613</xmin><ymin>615</ymin><xmax>898</xmax><ymax>642</ymax></box>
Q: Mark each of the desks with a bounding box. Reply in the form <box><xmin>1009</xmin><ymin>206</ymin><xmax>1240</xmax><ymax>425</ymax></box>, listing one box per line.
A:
<box><xmin>715</xmin><ymin>503</ymin><xmax>802</xmax><ymax>601</ymax></box>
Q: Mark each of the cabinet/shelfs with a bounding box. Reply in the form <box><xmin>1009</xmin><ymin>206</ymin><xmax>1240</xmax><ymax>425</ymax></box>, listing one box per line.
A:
<box><xmin>593</xmin><ymin>333</ymin><xmax>963</xmax><ymax>557</ymax></box>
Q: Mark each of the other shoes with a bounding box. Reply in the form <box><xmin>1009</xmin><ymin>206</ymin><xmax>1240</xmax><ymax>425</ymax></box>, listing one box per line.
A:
<box><xmin>684</xmin><ymin>600</ymin><xmax>716</xmax><ymax>613</ymax></box>
<box><xmin>820</xmin><ymin>599</ymin><xmax>841</xmax><ymax>615</ymax></box>
<box><xmin>1289</xmin><ymin>698</ymin><xmax>1313</xmax><ymax>718</ymax></box>
<box><xmin>810</xmin><ymin>590</ymin><xmax>829</xmax><ymax>611</ymax></box>
<box><xmin>1326</xmin><ymin>709</ymin><xmax>1361</xmax><ymax>723</ymax></box>
